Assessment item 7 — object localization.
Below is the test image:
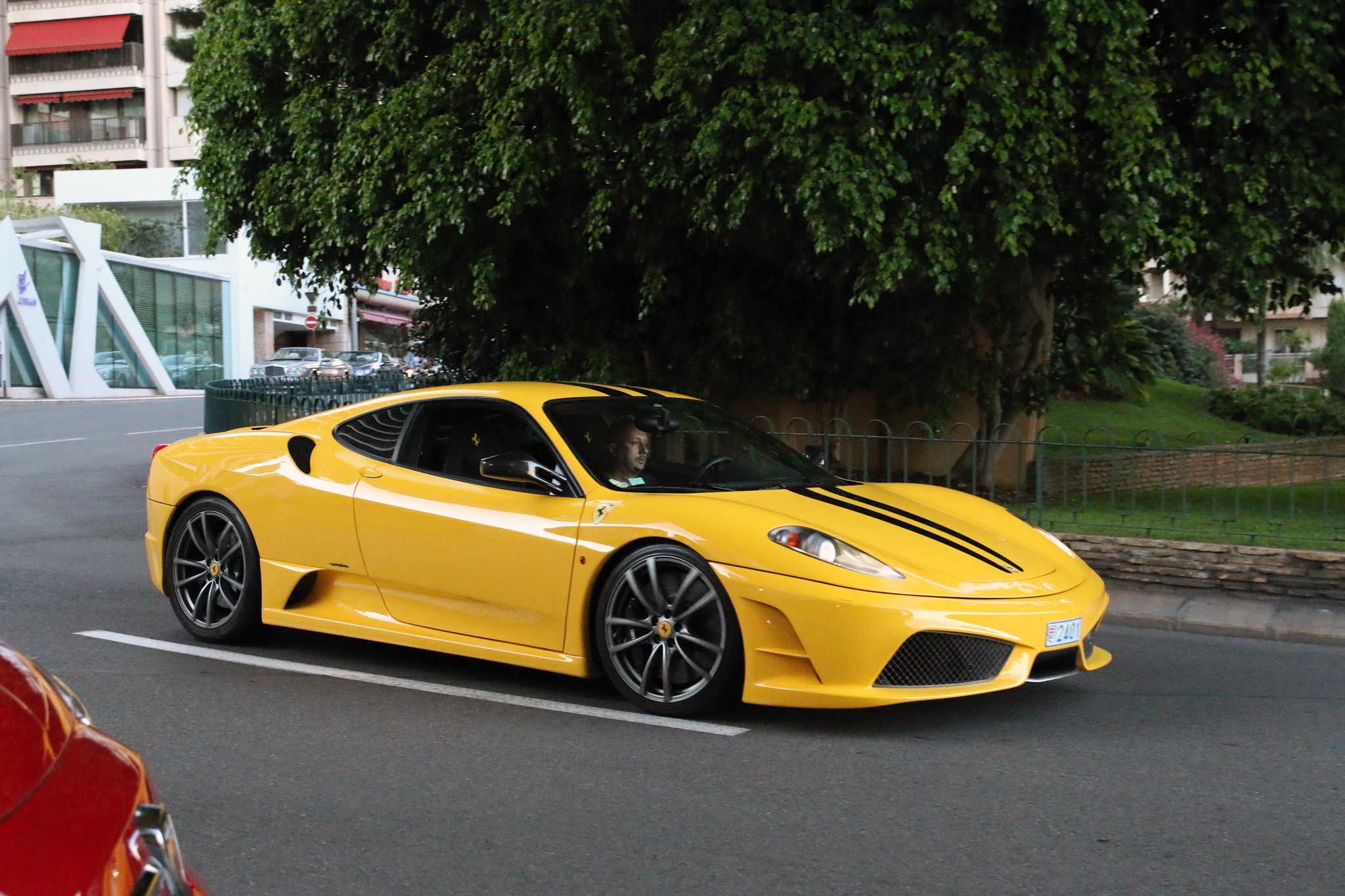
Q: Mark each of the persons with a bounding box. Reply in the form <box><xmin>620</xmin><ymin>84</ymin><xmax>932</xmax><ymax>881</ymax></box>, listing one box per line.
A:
<box><xmin>594</xmin><ymin>417</ymin><xmax>661</xmax><ymax>490</ymax></box>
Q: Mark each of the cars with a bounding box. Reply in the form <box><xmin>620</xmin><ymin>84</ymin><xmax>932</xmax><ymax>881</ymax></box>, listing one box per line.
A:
<box><xmin>1</xmin><ymin>643</ymin><xmax>212</xmax><ymax>896</ymax></box>
<box><xmin>92</xmin><ymin>349</ymin><xmax>225</xmax><ymax>389</ymax></box>
<box><xmin>334</xmin><ymin>350</ymin><xmax>444</xmax><ymax>394</ymax></box>
<box><xmin>249</xmin><ymin>346</ymin><xmax>355</xmax><ymax>394</ymax></box>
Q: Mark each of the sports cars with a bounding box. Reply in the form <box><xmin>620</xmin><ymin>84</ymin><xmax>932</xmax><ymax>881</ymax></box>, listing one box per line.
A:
<box><xmin>144</xmin><ymin>379</ymin><xmax>1113</xmax><ymax>718</ymax></box>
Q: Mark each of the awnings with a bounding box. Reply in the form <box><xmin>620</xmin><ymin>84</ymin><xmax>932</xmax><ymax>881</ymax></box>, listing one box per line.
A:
<box><xmin>13</xmin><ymin>86</ymin><xmax>135</xmax><ymax>104</ymax></box>
<box><xmin>3</xmin><ymin>12</ymin><xmax>132</xmax><ymax>55</ymax></box>
